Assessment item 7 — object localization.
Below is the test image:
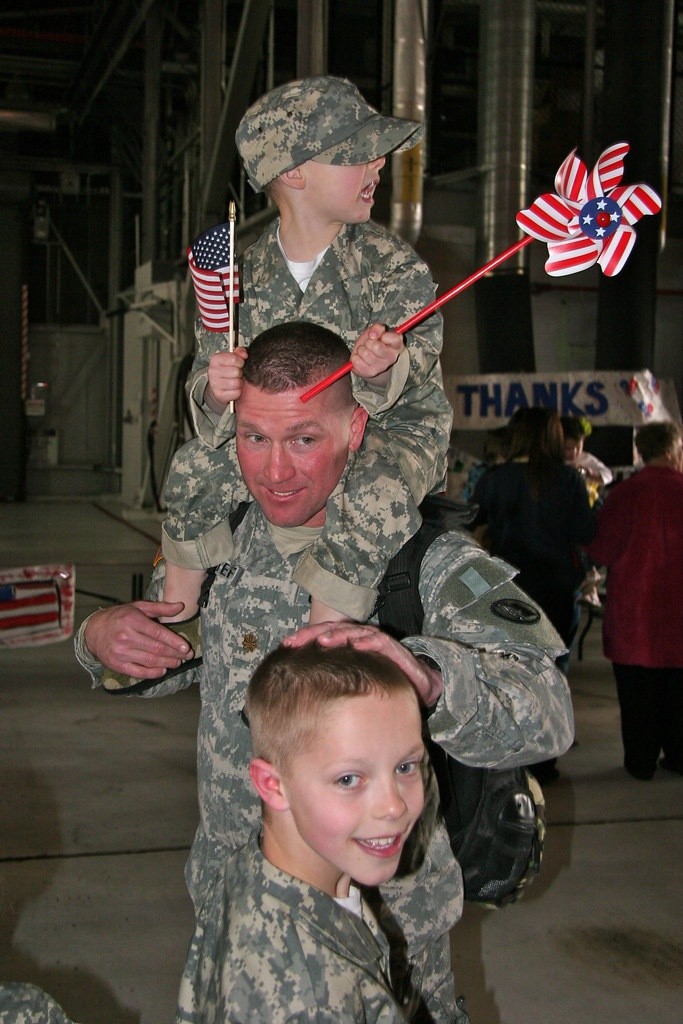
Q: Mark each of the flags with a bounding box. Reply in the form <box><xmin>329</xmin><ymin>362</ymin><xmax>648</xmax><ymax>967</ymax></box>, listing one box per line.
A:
<box><xmin>186</xmin><ymin>222</ymin><xmax>240</xmax><ymax>333</ymax></box>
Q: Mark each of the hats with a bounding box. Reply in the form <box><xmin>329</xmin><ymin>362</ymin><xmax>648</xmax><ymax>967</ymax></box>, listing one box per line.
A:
<box><xmin>236</xmin><ymin>76</ymin><xmax>425</xmax><ymax>194</ymax></box>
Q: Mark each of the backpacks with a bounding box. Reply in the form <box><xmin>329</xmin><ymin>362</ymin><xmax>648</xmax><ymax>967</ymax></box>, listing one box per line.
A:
<box><xmin>197</xmin><ymin>502</ymin><xmax>549</xmax><ymax>912</ymax></box>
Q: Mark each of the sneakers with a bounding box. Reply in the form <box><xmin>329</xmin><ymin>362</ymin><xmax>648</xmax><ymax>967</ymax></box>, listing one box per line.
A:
<box><xmin>102</xmin><ymin>607</ymin><xmax>203</xmax><ymax>695</ymax></box>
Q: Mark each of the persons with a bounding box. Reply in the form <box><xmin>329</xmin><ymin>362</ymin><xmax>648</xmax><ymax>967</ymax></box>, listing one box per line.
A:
<box><xmin>174</xmin><ymin>638</ymin><xmax>425</xmax><ymax>1024</ymax></box>
<box><xmin>580</xmin><ymin>422</ymin><xmax>683</xmax><ymax>781</ymax></box>
<box><xmin>102</xmin><ymin>76</ymin><xmax>454</xmax><ymax>696</ymax></box>
<box><xmin>462</xmin><ymin>407</ymin><xmax>614</xmax><ymax>683</ymax></box>
<box><xmin>74</xmin><ymin>322</ymin><xmax>575</xmax><ymax>1024</ymax></box>
